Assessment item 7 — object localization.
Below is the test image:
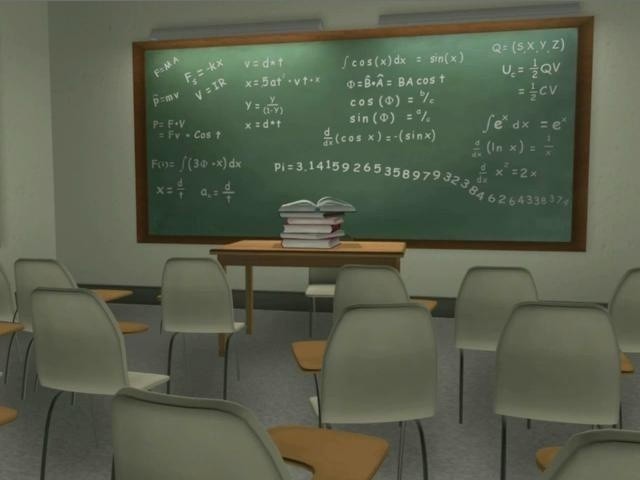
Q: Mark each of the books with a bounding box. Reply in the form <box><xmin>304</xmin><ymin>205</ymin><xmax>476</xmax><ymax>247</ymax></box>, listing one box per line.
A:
<box><xmin>278</xmin><ymin>195</ymin><xmax>359</xmax><ymax>248</ymax></box>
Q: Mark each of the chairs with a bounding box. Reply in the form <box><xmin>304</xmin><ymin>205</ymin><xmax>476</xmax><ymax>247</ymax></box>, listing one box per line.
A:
<box><xmin>535</xmin><ymin>429</ymin><xmax>640</xmax><ymax>480</ymax></box>
<box><xmin>292</xmin><ymin>304</ymin><xmax>435</xmax><ymax>480</ymax></box>
<box><xmin>608</xmin><ymin>268</ymin><xmax>640</xmax><ymax>373</ymax></box>
<box><xmin>1</xmin><ymin>257</ymin><xmax>150</xmax><ymax>425</ymax></box>
<box><xmin>482</xmin><ymin>300</ymin><xmax>622</xmax><ymax>480</ymax></box>
<box><xmin>160</xmin><ymin>256</ymin><xmax>246</xmax><ymax>401</ymax></box>
<box><xmin>33</xmin><ymin>286</ymin><xmax>171</xmax><ymax>479</ymax></box>
<box><xmin>305</xmin><ymin>264</ymin><xmax>438</xmax><ymax>339</ymax></box>
<box><xmin>455</xmin><ymin>266</ymin><xmax>539</xmax><ymax>430</ymax></box>
<box><xmin>110</xmin><ymin>389</ymin><xmax>390</xmax><ymax>480</ymax></box>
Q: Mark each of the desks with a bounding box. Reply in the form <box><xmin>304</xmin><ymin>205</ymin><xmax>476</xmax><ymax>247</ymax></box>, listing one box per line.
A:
<box><xmin>211</xmin><ymin>238</ymin><xmax>406</xmax><ymax>356</ymax></box>
